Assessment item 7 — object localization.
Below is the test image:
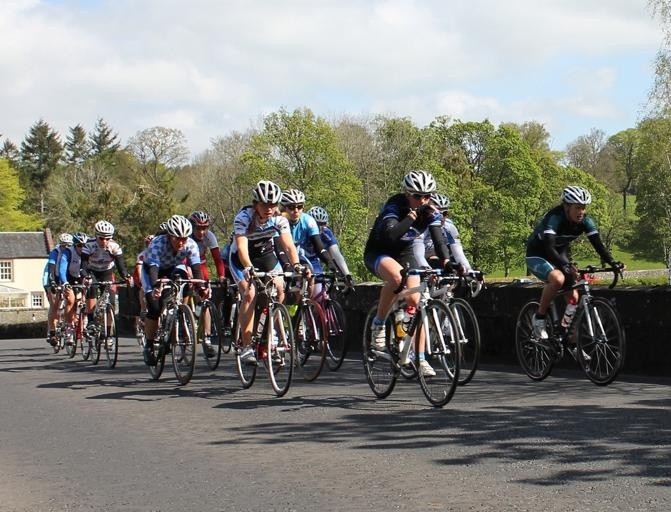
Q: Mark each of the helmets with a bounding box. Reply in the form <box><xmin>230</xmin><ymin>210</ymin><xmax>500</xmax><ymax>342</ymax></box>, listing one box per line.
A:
<box><xmin>144</xmin><ymin>234</ymin><xmax>155</xmax><ymax>243</ymax></box>
<box><xmin>93</xmin><ymin>219</ymin><xmax>115</xmax><ymax>239</ymax></box>
<box><xmin>306</xmin><ymin>205</ymin><xmax>329</xmax><ymax>223</ymax></box>
<box><xmin>158</xmin><ymin>222</ymin><xmax>168</xmax><ymax>233</ymax></box>
<box><xmin>252</xmin><ymin>180</ymin><xmax>283</xmax><ymax>205</ymax></box>
<box><xmin>72</xmin><ymin>232</ymin><xmax>88</xmax><ymax>246</ymax></box>
<box><xmin>560</xmin><ymin>186</ymin><xmax>592</xmax><ymax>204</ymax></box>
<box><xmin>191</xmin><ymin>210</ymin><xmax>210</xmax><ymax>227</ymax></box>
<box><xmin>402</xmin><ymin>170</ymin><xmax>437</xmax><ymax>195</ymax></box>
<box><xmin>282</xmin><ymin>188</ymin><xmax>305</xmax><ymax>206</ymax></box>
<box><xmin>431</xmin><ymin>192</ymin><xmax>450</xmax><ymax>212</ymax></box>
<box><xmin>58</xmin><ymin>233</ymin><xmax>73</xmax><ymax>245</ymax></box>
<box><xmin>167</xmin><ymin>214</ymin><xmax>193</xmax><ymax>238</ymax></box>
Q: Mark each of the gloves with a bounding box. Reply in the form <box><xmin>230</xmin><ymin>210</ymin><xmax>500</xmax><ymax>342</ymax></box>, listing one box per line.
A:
<box><xmin>408</xmin><ymin>204</ymin><xmax>431</xmax><ymax>220</ymax></box>
<box><xmin>124</xmin><ymin>274</ymin><xmax>134</xmax><ymax>287</ymax></box>
<box><xmin>61</xmin><ymin>282</ymin><xmax>71</xmax><ymax>292</ymax></box>
<box><xmin>344</xmin><ymin>273</ymin><xmax>355</xmax><ymax>287</ymax></box>
<box><xmin>325</xmin><ymin>264</ymin><xmax>338</xmax><ymax>279</ymax></box>
<box><xmin>466</xmin><ymin>270</ymin><xmax>486</xmax><ymax>282</ymax></box>
<box><xmin>241</xmin><ymin>266</ymin><xmax>259</xmax><ymax>277</ymax></box>
<box><xmin>444</xmin><ymin>261</ymin><xmax>464</xmax><ymax>273</ymax></box>
<box><xmin>602</xmin><ymin>259</ymin><xmax>624</xmax><ymax>269</ymax></box>
<box><xmin>292</xmin><ymin>263</ymin><xmax>310</xmax><ymax>274</ymax></box>
<box><xmin>50</xmin><ymin>281</ymin><xmax>58</xmax><ymax>295</ymax></box>
<box><xmin>82</xmin><ymin>276</ymin><xmax>92</xmax><ymax>288</ymax></box>
<box><xmin>562</xmin><ymin>263</ymin><xmax>573</xmax><ymax>277</ymax></box>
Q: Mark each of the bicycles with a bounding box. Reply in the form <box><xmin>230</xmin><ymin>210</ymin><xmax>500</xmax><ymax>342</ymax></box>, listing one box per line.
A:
<box><xmin>211</xmin><ymin>280</ymin><xmax>239</xmax><ymax>353</ymax></box>
<box><xmin>516</xmin><ymin>263</ymin><xmax>626</xmax><ymax>387</ymax></box>
<box><xmin>46</xmin><ymin>286</ymin><xmax>84</xmax><ymax>357</ymax></box>
<box><xmin>172</xmin><ymin>278</ymin><xmax>230</xmax><ymax>370</ymax></box>
<box><xmin>262</xmin><ymin>269</ymin><xmax>338</xmax><ymax>382</ymax></box>
<box><xmin>137</xmin><ymin>274</ymin><xmax>211</xmax><ymax>385</ymax></box>
<box><xmin>361</xmin><ymin>264</ymin><xmax>467</xmax><ymax>407</ymax></box>
<box><xmin>394</xmin><ymin>270</ymin><xmax>485</xmax><ymax>385</ymax></box>
<box><xmin>232</xmin><ymin>264</ymin><xmax>312</xmax><ymax>395</ymax></box>
<box><xmin>80</xmin><ymin>274</ymin><xmax>132</xmax><ymax>367</ymax></box>
<box><xmin>62</xmin><ymin>280</ymin><xmax>96</xmax><ymax>360</ymax></box>
<box><xmin>290</xmin><ymin>273</ymin><xmax>354</xmax><ymax>372</ymax></box>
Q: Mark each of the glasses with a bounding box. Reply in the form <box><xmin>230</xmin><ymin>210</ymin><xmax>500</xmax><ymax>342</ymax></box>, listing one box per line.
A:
<box><xmin>288</xmin><ymin>205</ymin><xmax>303</xmax><ymax>210</ymax></box>
<box><xmin>413</xmin><ymin>193</ymin><xmax>431</xmax><ymax>200</ymax></box>
<box><xmin>316</xmin><ymin>221</ymin><xmax>327</xmax><ymax>225</ymax></box>
<box><xmin>76</xmin><ymin>244</ymin><xmax>85</xmax><ymax>247</ymax></box>
<box><xmin>442</xmin><ymin>211</ymin><xmax>449</xmax><ymax>217</ymax></box>
<box><xmin>98</xmin><ymin>236</ymin><xmax>111</xmax><ymax>241</ymax></box>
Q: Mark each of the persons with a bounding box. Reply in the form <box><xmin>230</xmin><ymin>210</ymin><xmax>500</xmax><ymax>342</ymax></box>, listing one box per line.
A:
<box><xmin>280</xmin><ymin>188</ymin><xmax>340</xmax><ymax>274</ymax></box>
<box><xmin>307</xmin><ymin>205</ymin><xmax>355</xmax><ymax>302</ymax></box>
<box><xmin>80</xmin><ymin>220</ymin><xmax>135</xmax><ymax>351</ymax></box>
<box><xmin>134</xmin><ymin>235</ymin><xmax>156</xmax><ymax>338</ymax></box>
<box><xmin>55</xmin><ymin>231</ymin><xmax>89</xmax><ymax>346</ymax></box>
<box><xmin>43</xmin><ymin>233</ymin><xmax>74</xmax><ymax>346</ymax></box>
<box><xmin>228</xmin><ymin>179</ymin><xmax>311</xmax><ymax>365</ymax></box>
<box><xmin>142</xmin><ymin>213</ymin><xmax>212</xmax><ymax>366</ymax></box>
<box><xmin>363</xmin><ymin>170</ymin><xmax>452</xmax><ymax>377</ymax></box>
<box><xmin>526</xmin><ymin>186</ymin><xmax>626</xmax><ymax>360</ymax></box>
<box><xmin>187</xmin><ymin>209</ymin><xmax>226</xmax><ymax>357</ymax></box>
<box><xmin>414</xmin><ymin>193</ymin><xmax>486</xmax><ymax>337</ymax></box>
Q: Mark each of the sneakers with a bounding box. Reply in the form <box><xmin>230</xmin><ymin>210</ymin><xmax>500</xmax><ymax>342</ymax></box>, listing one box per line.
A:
<box><xmin>271</xmin><ymin>353</ymin><xmax>282</xmax><ymax>363</ymax></box>
<box><xmin>224</xmin><ymin>325</ymin><xmax>232</xmax><ymax>337</ymax></box>
<box><xmin>202</xmin><ymin>336</ymin><xmax>216</xmax><ymax>358</ymax></box>
<box><xmin>106</xmin><ymin>337</ymin><xmax>114</xmax><ymax>351</ymax></box>
<box><xmin>371</xmin><ymin>319</ymin><xmax>388</xmax><ymax>351</ymax></box>
<box><xmin>567</xmin><ymin>346</ymin><xmax>592</xmax><ymax>361</ymax></box>
<box><xmin>240</xmin><ymin>345</ymin><xmax>257</xmax><ymax>364</ymax></box>
<box><xmin>136</xmin><ymin>320</ymin><xmax>146</xmax><ymax>337</ymax></box>
<box><xmin>531</xmin><ymin>313</ymin><xmax>549</xmax><ymax>340</ymax></box>
<box><xmin>63</xmin><ymin>329</ymin><xmax>74</xmax><ymax>345</ymax></box>
<box><xmin>143</xmin><ymin>346</ymin><xmax>158</xmax><ymax>366</ymax></box>
<box><xmin>419</xmin><ymin>359</ymin><xmax>437</xmax><ymax>376</ymax></box>
